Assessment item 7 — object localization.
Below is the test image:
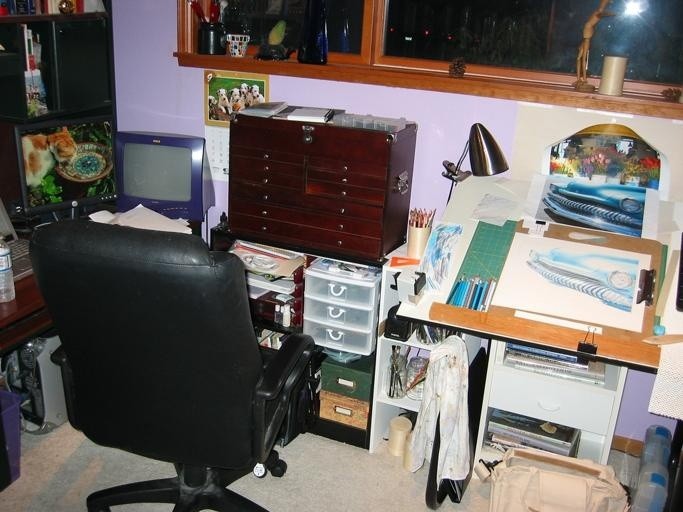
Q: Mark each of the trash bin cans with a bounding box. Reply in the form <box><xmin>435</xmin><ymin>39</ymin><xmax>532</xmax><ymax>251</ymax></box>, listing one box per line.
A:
<box><xmin>0</xmin><ymin>390</ymin><xmax>22</xmax><ymax>491</ymax></box>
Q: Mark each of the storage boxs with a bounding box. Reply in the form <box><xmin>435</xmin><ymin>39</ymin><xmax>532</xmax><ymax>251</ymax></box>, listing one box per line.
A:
<box><xmin>314</xmin><ymin>387</ymin><xmax>371</xmax><ymax>430</ymax></box>
<box><xmin>320</xmin><ymin>353</ymin><xmax>376</xmax><ymax>402</ymax></box>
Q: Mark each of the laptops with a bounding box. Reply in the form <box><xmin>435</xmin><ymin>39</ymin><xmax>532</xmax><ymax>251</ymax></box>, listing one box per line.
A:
<box><xmin>0</xmin><ymin>197</ymin><xmax>33</xmax><ymax>282</ymax></box>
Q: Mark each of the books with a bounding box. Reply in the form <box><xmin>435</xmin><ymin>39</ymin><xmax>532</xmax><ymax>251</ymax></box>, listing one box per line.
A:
<box><xmin>289</xmin><ymin>107</ymin><xmax>334</xmax><ymax>123</ymax></box>
<box><xmin>87</xmin><ymin>202</ymin><xmax>193</xmax><ymax>235</ymax></box>
<box><xmin>485</xmin><ymin>339</ymin><xmax>604</xmax><ymax>459</ymax></box>
<box><xmin>227</xmin><ymin>239</ymin><xmax>303</xmax><ymax>300</ymax></box>
<box><xmin>0</xmin><ymin>1</ymin><xmax>105</xmax><ymax>117</ymax></box>
<box><xmin>257</xmin><ymin>329</ymin><xmax>283</xmax><ymax>351</ymax></box>
<box><xmin>237</xmin><ymin>101</ymin><xmax>287</xmax><ymax>118</ymax></box>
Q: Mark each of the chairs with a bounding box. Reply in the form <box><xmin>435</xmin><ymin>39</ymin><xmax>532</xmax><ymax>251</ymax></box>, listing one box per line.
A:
<box><xmin>25</xmin><ymin>219</ymin><xmax>325</xmax><ymax>511</ymax></box>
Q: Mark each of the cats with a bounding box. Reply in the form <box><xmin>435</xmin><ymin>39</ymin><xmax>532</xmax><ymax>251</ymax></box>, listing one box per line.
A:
<box><xmin>21</xmin><ymin>126</ymin><xmax>78</xmax><ymax>188</ymax></box>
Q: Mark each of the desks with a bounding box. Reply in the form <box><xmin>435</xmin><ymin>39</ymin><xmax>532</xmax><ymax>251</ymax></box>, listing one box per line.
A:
<box><xmin>1</xmin><ymin>258</ymin><xmax>55</xmax><ymax>363</ymax></box>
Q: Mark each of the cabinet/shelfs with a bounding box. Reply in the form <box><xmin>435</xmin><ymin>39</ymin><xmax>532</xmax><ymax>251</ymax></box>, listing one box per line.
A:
<box><xmin>302</xmin><ymin>258</ymin><xmax>382</xmax><ymax>358</ymax></box>
<box><xmin>226</xmin><ymin>103</ymin><xmax>417</xmax><ymax>261</ymax></box>
<box><xmin>368</xmin><ymin>249</ymin><xmax>484</xmax><ymax>465</ymax></box>
<box><xmin>475</xmin><ymin>324</ymin><xmax>631</xmax><ymax>489</ymax></box>
<box><xmin>0</xmin><ymin>1</ymin><xmax>119</xmax><ymax>239</ymax></box>
<box><xmin>207</xmin><ymin>220</ymin><xmax>397</xmax><ymax>455</ymax></box>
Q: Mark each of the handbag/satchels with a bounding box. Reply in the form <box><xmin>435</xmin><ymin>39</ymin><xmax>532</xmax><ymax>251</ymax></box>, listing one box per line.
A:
<box><xmin>474</xmin><ymin>447</ymin><xmax>631</xmax><ymax>511</ymax></box>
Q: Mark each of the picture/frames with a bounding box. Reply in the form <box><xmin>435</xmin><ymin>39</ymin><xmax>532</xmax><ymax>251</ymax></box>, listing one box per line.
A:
<box><xmin>201</xmin><ymin>67</ymin><xmax>270</xmax><ymax>129</ymax></box>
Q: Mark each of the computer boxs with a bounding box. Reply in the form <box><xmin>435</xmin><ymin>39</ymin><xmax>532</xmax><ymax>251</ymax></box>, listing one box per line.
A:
<box><xmin>0</xmin><ymin>327</ymin><xmax>69</xmax><ymax>435</ymax></box>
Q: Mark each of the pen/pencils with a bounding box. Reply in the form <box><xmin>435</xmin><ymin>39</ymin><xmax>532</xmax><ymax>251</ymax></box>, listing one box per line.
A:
<box><xmin>389</xmin><ymin>345</ymin><xmax>411</xmax><ymax>398</ymax></box>
<box><xmin>409</xmin><ymin>208</ymin><xmax>437</xmax><ymax>227</ymax></box>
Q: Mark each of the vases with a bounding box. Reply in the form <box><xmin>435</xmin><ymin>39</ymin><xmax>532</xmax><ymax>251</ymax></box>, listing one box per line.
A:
<box><xmin>599</xmin><ymin>54</ymin><xmax>627</xmax><ymax>96</ymax></box>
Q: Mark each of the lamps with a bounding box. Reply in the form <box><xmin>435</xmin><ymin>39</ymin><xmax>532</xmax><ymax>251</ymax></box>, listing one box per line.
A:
<box><xmin>440</xmin><ymin>116</ymin><xmax>510</xmax><ymax>206</ymax></box>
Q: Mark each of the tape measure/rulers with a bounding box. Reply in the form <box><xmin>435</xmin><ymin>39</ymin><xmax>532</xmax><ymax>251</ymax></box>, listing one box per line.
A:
<box><xmin>389</xmin><ymin>254</ymin><xmax>420</xmax><ymax>268</ymax></box>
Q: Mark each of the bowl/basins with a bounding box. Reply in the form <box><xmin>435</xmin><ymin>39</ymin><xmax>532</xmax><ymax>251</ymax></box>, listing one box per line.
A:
<box><xmin>56</xmin><ymin>141</ymin><xmax>113</xmax><ymax>184</ymax></box>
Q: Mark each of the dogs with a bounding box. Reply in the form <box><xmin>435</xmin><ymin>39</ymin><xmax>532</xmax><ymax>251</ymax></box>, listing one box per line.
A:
<box><xmin>208</xmin><ymin>83</ymin><xmax>265</xmax><ymax>120</ymax></box>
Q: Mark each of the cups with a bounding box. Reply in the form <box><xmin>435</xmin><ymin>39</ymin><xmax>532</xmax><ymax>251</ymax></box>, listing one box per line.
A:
<box><xmin>385</xmin><ymin>414</ymin><xmax>412</xmax><ymax>458</ymax></box>
<box><xmin>199</xmin><ymin>21</ymin><xmax>224</xmax><ymax>55</ymax></box>
<box><xmin>227</xmin><ymin>34</ymin><xmax>251</xmax><ymax>60</ymax></box>
<box><xmin>405</xmin><ymin>225</ymin><xmax>431</xmax><ymax>260</ymax></box>
<box><xmin>597</xmin><ymin>54</ymin><xmax>629</xmax><ymax>97</ymax></box>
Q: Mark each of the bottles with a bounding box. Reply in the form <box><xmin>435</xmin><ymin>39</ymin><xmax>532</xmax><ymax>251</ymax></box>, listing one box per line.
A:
<box><xmin>406</xmin><ymin>356</ymin><xmax>425</xmax><ymax>403</ymax></box>
<box><xmin>330</xmin><ymin>1</ymin><xmax>353</xmax><ymax>54</ymax></box>
<box><xmin>0</xmin><ymin>233</ymin><xmax>17</xmax><ymax>305</ymax></box>
<box><xmin>384</xmin><ymin>355</ymin><xmax>406</xmax><ymax>398</ymax></box>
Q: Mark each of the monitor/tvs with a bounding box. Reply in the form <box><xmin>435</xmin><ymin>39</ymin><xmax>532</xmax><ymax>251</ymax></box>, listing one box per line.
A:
<box><xmin>15</xmin><ymin>115</ymin><xmax>118</xmax><ymax>222</ymax></box>
<box><xmin>114</xmin><ymin>131</ymin><xmax>205</xmax><ymax>223</ymax></box>
